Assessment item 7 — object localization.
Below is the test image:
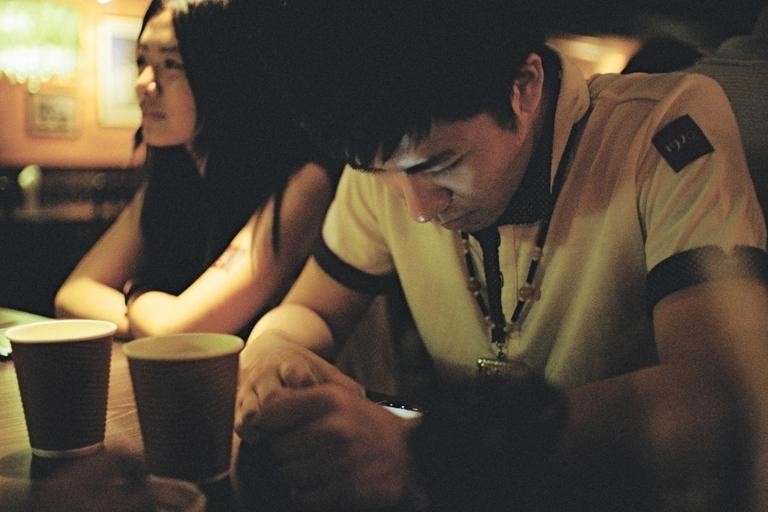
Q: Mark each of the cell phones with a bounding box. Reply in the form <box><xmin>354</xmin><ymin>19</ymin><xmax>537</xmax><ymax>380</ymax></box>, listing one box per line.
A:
<box><xmin>376</xmin><ymin>397</ymin><xmax>433</xmax><ymax>420</ymax></box>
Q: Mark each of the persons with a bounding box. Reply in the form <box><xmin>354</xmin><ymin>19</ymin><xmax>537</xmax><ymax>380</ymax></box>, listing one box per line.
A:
<box><xmin>680</xmin><ymin>3</ymin><xmax>768</xmax><ymax>232</ymax></box>
<box><xmin>52</xmin><ymin>0</ymin><xmax>334</xmax><ymax>341</ymax></box>
<box><xmin>231</xmin><ymin>1</ymin><xmax>767</xmax><ymax>512</ymax></box>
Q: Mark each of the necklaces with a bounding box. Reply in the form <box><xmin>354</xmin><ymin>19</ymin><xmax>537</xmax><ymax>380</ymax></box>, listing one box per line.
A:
<box><xmin>460</xmin><ymin>107</ymin><xmax>583</xmax><ymax>381</ymax></box>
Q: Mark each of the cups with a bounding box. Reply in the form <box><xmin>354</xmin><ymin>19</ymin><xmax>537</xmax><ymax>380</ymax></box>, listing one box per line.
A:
<box><xmin>120</xmin><ymin>331</ymin><xmax>242</xmax><ymax>479</ymax></box>
<box><xmin>3</xmin><ymin>313</ymin><xmax>119</xmax><ymax>465</ymax></box>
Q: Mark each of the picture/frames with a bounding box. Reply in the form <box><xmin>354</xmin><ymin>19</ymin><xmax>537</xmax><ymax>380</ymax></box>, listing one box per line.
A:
<box><xmin>23</xmin><ymin>11</ymin><xmax>145</xmax><ymax>140</ymax></box>
